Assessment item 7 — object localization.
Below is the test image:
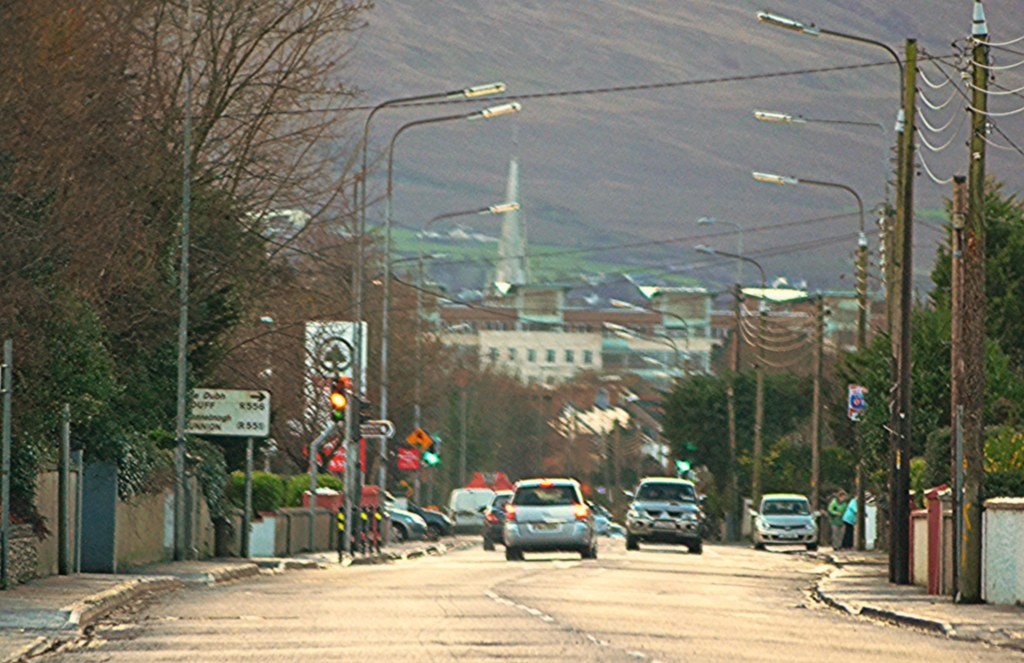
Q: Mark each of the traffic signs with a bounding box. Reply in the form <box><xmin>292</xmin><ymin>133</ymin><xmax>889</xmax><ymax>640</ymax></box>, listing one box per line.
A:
<box><xmin>184</xmin><ymin>387</ymin><xmax>271</xmax><ymax>437</ymax></box>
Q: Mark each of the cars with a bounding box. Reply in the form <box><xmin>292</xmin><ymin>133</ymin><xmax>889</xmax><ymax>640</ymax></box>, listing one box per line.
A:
<box><xmin>502</xmin><ymin>477</ymin><xmax>602</xmax><ymax>562</ymax></box>
<box><xmin>476</xmin><ymin>490</ymin><xmax>516</xmax><ymax>552</ymax></box>
<box><xmin>404</xmin><ymin>500</ymin><xmax>456</xmax><ymax>542</ymax></box>
<box><xmin>382</xmin><ymin>504</ymin><xmax>430</xmax><ymax>544</ymax></box>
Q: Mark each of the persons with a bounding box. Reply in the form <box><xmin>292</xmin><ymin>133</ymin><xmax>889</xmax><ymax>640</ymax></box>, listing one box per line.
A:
<box><xmin>827</xmin><ymin>490</ymin><xmax>865</xmax><ymax>550</ymax></box>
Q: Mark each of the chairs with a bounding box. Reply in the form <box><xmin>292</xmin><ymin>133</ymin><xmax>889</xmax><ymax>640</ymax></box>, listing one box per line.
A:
<box><xmin>794</xmin><ymin>504</ymin><xmax>802</xmax><ymax>515</ymax></box>
<box><xmin>766</xmin><ymin>505</ymin><xmax>779</xmax><ymax>514</ymax></box>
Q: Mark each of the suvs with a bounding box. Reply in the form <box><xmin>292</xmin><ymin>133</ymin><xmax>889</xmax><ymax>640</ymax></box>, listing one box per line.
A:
<box><xmin>625</xmin><ymin>477</ymin><xmax>704</xmax><ymax>554</ymax></box>
<box><xmin>747</xmin><ymin>493</ymin><xmax>820</xmax><ymax>554</ymax></box>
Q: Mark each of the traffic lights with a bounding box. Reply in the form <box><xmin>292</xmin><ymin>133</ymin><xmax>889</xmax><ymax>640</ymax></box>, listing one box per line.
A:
<box><xmin>328</xmin><ymin>391</ymin><xmax>347</xmax><ymax>423</ymax></box>
<box><xmin>426</xmin><ymin>441</ymin><xmax>442</xmax><ymax>469</ymax></box>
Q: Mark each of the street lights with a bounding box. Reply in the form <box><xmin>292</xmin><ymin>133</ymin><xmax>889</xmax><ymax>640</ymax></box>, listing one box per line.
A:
<box><xmin>376</xmin><ymin>202</ymin><xmax>521</xmax><ymax>488</ymax></box>
<box><xmin>372</xmin><ymin>101</ymin><xmax>525</xmax><ymax>489</ymax></box>
<box><xmin>747</xmin><ymin>108</ymin><xmax>893</xmax><ymax>345</ymax></box>
<box><xmin>752</xmin><ymin>4</ymin><xmax>916</xmax><ymax>583</ymax></box>
<box><xmin>750</xmin><ymin>169</ymin><xmax>868</xmax><ymax>552</ymax></box>
<box><xmin>339</xmin><ymin>79</ymin><xmax>508</xmax><ymax>560</ymax></box>
